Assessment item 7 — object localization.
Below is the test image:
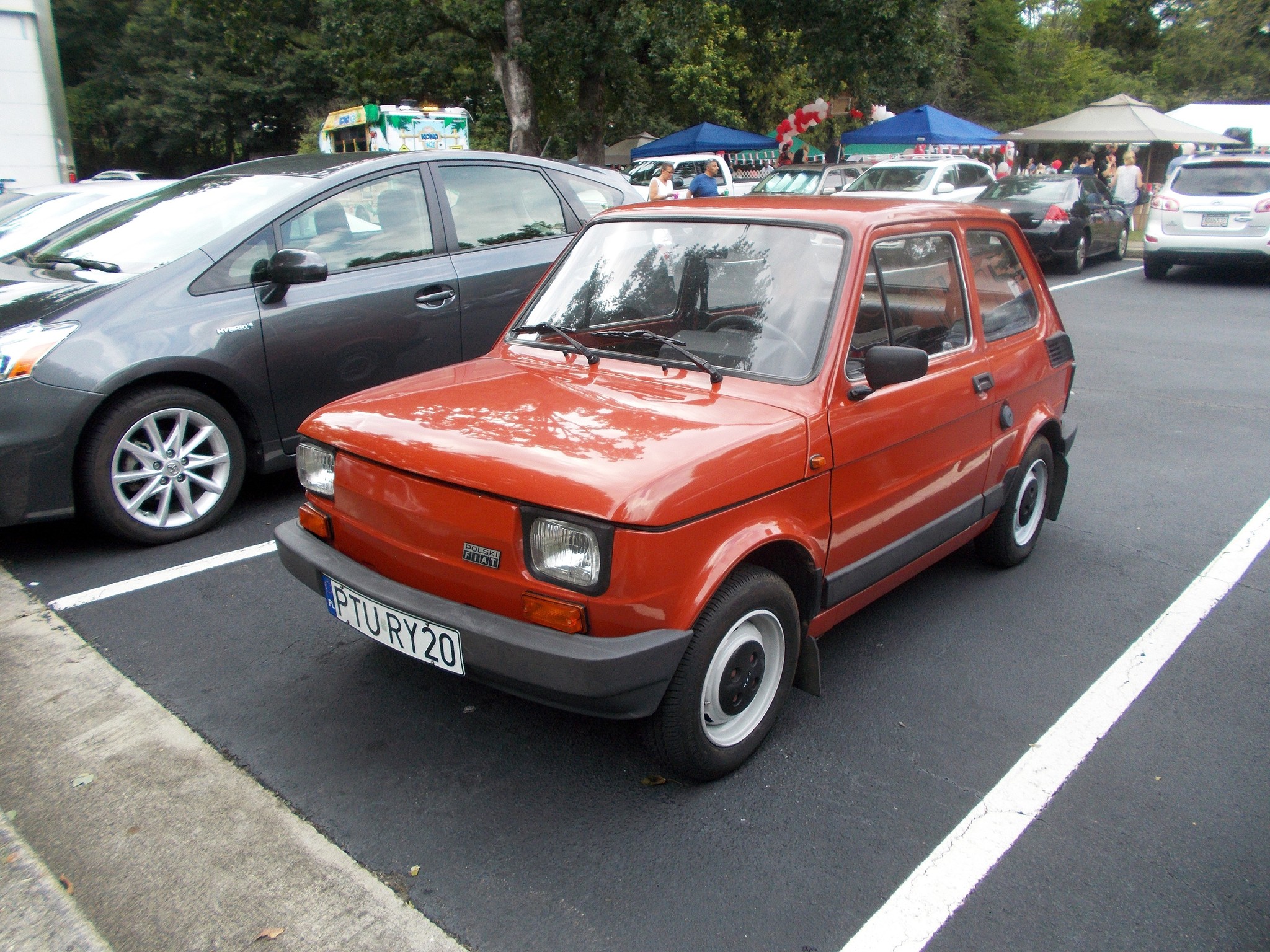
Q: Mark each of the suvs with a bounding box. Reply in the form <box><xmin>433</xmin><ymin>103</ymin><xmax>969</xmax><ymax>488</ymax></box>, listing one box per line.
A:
<box><xmin>1143</xmin><ymin>149</ymin><xmax>1270</xmax><ymax>279</ymax></box>
<box><xmin>829</xmin><ymin>153</ymin><xmax>998</xmax><ymax>203</ymax></box>
<box><xmin>271</xmin><ymin>195</ymin><xmax>1082</xmax><ymax>789</ymax></box>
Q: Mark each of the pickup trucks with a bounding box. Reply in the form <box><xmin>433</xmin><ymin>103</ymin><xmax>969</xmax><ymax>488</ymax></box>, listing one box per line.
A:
<box><xmin>577</xmin><ymin>154</ymin><xmax>766</xmax><ymax>223</ymax></box>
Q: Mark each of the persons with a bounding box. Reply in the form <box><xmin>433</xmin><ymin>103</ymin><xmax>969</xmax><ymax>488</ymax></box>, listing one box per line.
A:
<box><xmin>1069</xmin><ymin>143</ymin><xmax>1146</xmax><ymax>216</ymax></box>
<box><xmin>761</xmin><ymin>160</ymin><xmax>774</xmax><ymax>179</ymax></box>
<box><xmin>974</xmin><ymin>156</ymin><xmax>1058</xmax><ymax>181</ymax></box>
<box><xmin>825</xmin><ymin>138</ymin><xmax>846</xmax><ymax>163</ymax></box>
<box><xmin>729</xmin><ymin>162</ymin><xmax>734</xmax><ymax>174</ymax></box>
<box><xmin>648</xmin><ymin>162</ymin><xmax>679</xmax><ymax>201</ymax></box>
<box><xmin>686</xmin><ymin>158</ymin><xmax>729</xmax><ymax>199</ymax></box>
<box><xmin>1165</xmin><ymin>143</ymin><xmax>1196</xmax><ymax>179</ymax></box>
<box><xmin>775</xmin><ymin>143</ymin><xmax>810</xmax><ymax>168</ymax></box>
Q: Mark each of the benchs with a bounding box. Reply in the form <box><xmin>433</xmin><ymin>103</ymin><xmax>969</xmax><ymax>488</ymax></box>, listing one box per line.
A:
<box><xmin>855</xmin><ymin>298</ymin><xmax>959</xmax><ymax>334</ymax></box>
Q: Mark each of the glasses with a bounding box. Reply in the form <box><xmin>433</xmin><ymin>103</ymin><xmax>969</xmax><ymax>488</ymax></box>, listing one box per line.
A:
<box><xmin>705</xmin><ymin>158</ymin><xmax>718</xmax><ymax>166</ymax></box>
<box><xmin>664</xmin><ymin>169</ymin><xmax>674</xmax><ymax>174</ymax></box>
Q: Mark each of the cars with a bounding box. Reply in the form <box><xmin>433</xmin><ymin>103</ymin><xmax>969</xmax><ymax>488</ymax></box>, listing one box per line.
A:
<box><xmin>741</xmin><ymin>163</ymin><xmax>872</xmax><ymax>198</ymax></box>
<box><xmin>78</xmin><ymin>170</ymin><xmax>157</xmax><ymax>183</ymax></box>
<box><xmin>0</xmin><ymin>151</ymin><xmax>651</xmax><ymax>550</ymax></box>
<box><xmin>0</xmin><ymin>182</ymin><xmax>187</xmax><ymax>255</ymax></box>
<box><xmin>964</xmin><ymin>173</ymin><xmax>1130</xmax><ymax>275</ymax></box>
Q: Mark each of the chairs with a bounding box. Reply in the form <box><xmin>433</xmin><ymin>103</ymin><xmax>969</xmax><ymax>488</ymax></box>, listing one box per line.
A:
<box><xmin>306</xmin><ymin>202</ymin><xmax>352</xmax><ymax>251</ymax></box>
<box><xmin>373</xmin><ymin>186</ymin><xmax>415</xmax><ymax>237</ymax></box>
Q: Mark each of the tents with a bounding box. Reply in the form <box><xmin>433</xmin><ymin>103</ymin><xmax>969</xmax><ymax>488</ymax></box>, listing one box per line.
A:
<box><xmin>630</xmin><ymin>122</ymin><xmax>779</xmax><ymax>164</ymax></box>
<box><xmin>1162</xmin><ymin>104</ymin><xmax>1270</xmax><ymax>148</ymax></box>
<box><xmin>842</xmin><ymin>105</ymin><xmax>1008</xmax><ymax>162</ymax></box>
<box><xmin>731</xmin><ymin>130</ymin><xmax>827</xmax><ymax>163</ymax></box>
<box><xmin>989</xmin><ymin>93</ymin><xmax>1244</xmax><ymax>232</ymax></box>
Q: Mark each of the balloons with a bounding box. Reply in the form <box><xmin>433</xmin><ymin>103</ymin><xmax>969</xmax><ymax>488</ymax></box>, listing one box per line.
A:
<box><xmin>776</xmin><ymin>97</ymin><xmax>896</xmax><ymax>151</ymax></box>
<box><xmin>903</xmin><ymin>144</ymin><xmax>926</xmax><ymax>158</ymax></box>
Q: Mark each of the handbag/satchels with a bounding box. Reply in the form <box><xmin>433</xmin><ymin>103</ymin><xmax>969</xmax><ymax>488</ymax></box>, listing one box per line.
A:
<box><xmin>1136</xmin><ymin>185</ymin><xmax>1151</xmax><ymax>205</ymax></box>
